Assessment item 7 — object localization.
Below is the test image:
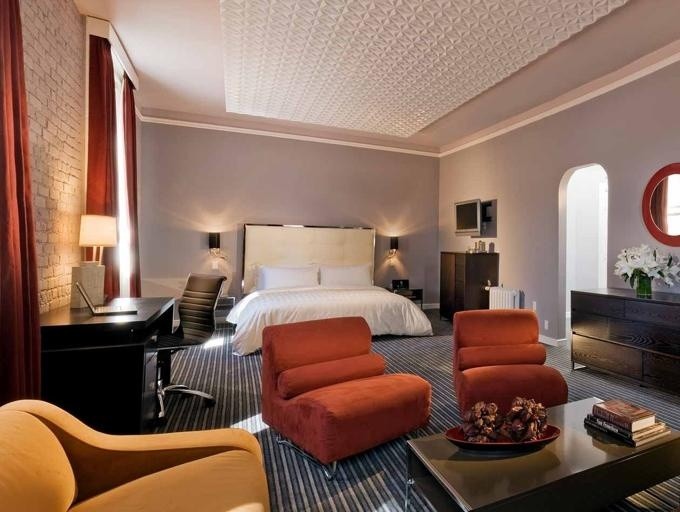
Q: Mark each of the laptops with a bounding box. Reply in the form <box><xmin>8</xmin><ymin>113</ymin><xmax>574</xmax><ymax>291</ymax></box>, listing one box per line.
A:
<box><xmin>75</xmin><ymin>279</ymin><xmax>138</xmax><ymax>316</ymax></box>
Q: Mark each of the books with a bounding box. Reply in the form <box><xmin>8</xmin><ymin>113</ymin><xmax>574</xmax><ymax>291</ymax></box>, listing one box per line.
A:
<box><xmin>581</xmin><ymin>397</ymin><xmax>674</xmax><ymax>451</ymax></box>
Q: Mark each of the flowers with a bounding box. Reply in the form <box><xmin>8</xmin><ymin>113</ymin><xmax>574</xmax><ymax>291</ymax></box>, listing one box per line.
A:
<box><xmin>612</xmin><ymin>245</ymin><xmax>680</xmax><ymax>288</ymax></box>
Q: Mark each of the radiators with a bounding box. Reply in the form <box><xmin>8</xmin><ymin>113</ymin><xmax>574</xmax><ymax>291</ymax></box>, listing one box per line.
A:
<box><xmin>487</xmin><ymin>286</ymin><xmax>520</xmax><ymax>308</ymax></box>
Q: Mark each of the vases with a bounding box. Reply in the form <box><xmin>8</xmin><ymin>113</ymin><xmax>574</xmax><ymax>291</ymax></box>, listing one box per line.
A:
<box><xmin>634</xmin><ymin>275</ymin><xmax>652</xmax><ymax>299</ymax></box>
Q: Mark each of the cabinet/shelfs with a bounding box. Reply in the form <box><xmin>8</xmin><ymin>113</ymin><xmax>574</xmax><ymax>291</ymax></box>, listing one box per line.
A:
<box><xmin>569</xmin><ymin>288</ymin><xmax>680</xmax><ymax>399</ymax></box>
<box><xmin>439</xmin><ymin>252</ymin><xmax>500</xmax><ymax>323</ymax></box>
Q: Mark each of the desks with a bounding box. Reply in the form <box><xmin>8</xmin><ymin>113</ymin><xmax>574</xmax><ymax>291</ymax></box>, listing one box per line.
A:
<box><xmin>35</xmin><ymin>296</ymin><xmax>175</xmax><ymax>432</ymax></box>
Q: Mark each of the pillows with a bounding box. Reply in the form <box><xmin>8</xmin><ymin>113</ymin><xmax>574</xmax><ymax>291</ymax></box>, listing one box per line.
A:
<box><xmin>254</xmin><ymin>261</ymin><xmax>373</xmax><ymax>291</ymax></box>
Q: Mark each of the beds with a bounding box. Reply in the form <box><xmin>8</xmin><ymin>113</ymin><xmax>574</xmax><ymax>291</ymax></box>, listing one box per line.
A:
<box><xmin>222</xmin><ymin>222</ymin><xmax>433</xmax><ymax>358</ymax></box>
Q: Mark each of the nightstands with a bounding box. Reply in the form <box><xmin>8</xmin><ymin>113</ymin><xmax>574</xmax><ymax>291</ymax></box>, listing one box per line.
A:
<box><xmin>214</xmin><ymin>297</ymin><xmax>238</xmax><ymax>334</ymax></box>
<box><xmin>390</xmin><ymin>289</ymin><xmax>424</xmax><ymax>311</ymax></box>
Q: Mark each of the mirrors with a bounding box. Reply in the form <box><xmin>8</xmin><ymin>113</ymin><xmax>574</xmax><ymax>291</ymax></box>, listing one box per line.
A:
<box><xmin>642</xmin><ymin>162</ymin><xmax>680</xmax><ymax>247</ymax></box>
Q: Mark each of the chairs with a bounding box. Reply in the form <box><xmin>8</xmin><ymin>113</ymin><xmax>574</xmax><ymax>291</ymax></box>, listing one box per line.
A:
<box><xmin>146</xmin><ymin>272</ymin><xmax>228</xmax><ymax>423</ymax></box>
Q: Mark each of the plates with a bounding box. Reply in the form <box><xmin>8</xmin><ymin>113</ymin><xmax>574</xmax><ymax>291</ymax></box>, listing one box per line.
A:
<box><xmin>444</xmin><ymin>424</ymin><xmax>563</xmax><ymax>457</ymax></box>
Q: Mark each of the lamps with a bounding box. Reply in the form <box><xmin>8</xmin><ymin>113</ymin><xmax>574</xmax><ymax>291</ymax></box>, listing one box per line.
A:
<box><xmin>206</xmin><ymin>232</ymin><xmax>222</xmax><ymax>259</ymax></box>
<box><xmin>70</xmin><ymin>215</ymin><xmax>119</xmax><ymax>309</ymax></box>
<box><xmin>387</xmin><ymin>235</ymin><xmax>399</xmax><ymax>257</ymax></box>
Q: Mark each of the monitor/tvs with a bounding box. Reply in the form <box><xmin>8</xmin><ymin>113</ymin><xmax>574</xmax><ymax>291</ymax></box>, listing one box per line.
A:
<box><xmin>454</xmin><ymin>198</ymin><xmax>482</xmax><ymax>237</ymax></box>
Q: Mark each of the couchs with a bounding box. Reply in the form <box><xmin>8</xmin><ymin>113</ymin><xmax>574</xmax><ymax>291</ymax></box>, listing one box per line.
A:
<box><xmin>257</xmin><ymin>313</ymin><xmax>434</xmax><ymax>483</ymax></box>
<box><xmin>1</xmin><ymin>397</ymin><xmax>274</xmax><ymax>511</ymax></box>
<box><xmin>452</xmin><ymin>307</ymin><xmax>568</xmax><ymax>420</ymax></box>
